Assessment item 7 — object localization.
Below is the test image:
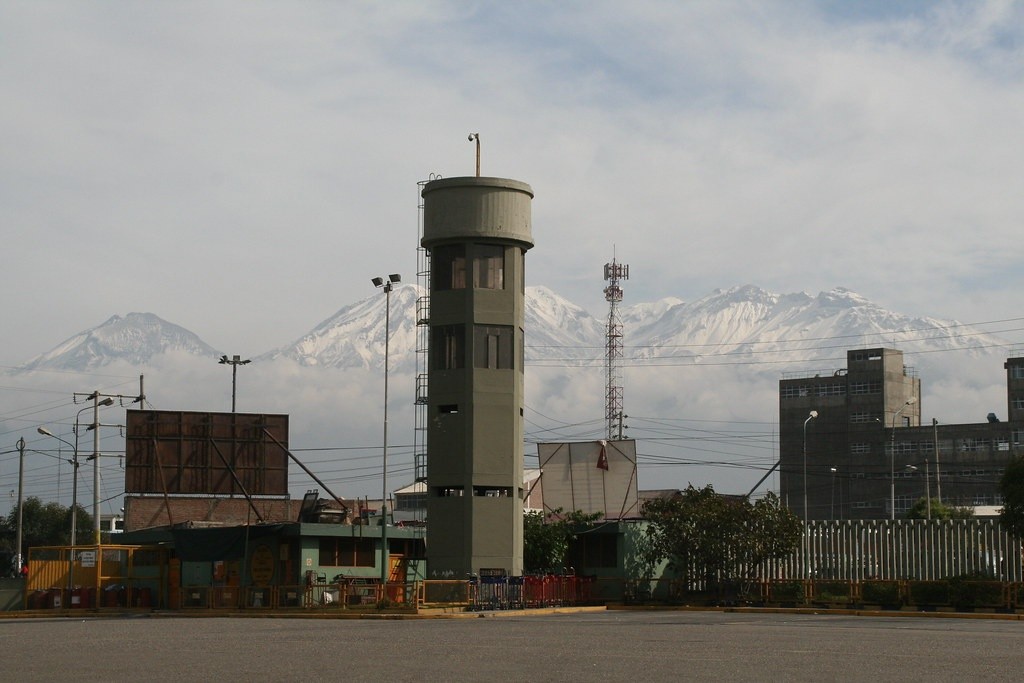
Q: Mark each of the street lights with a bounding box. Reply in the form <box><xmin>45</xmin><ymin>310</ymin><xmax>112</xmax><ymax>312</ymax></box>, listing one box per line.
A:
<box><xmin>889</xmin><ymin>397</ymin><xmax>919</xmax><ymax>520</ymax></box>
<box><xmin>37</xmin><ymin>396</ymin><xmax>115</xmax><ymax>608</ymax></box>
<box><xmin>218</xmin><ymin>352</ymin><xmax>252</xmax><ymax>499</ymax></box>
<box><xmin>802</xmin><ymin>410</ymin><xmax>818</xmax><ymax>595</ymax></box>
<box><xmin>372</xmin><ymin>272</ymin><xmax>400</xmax><ymax>583</ymax></box>
